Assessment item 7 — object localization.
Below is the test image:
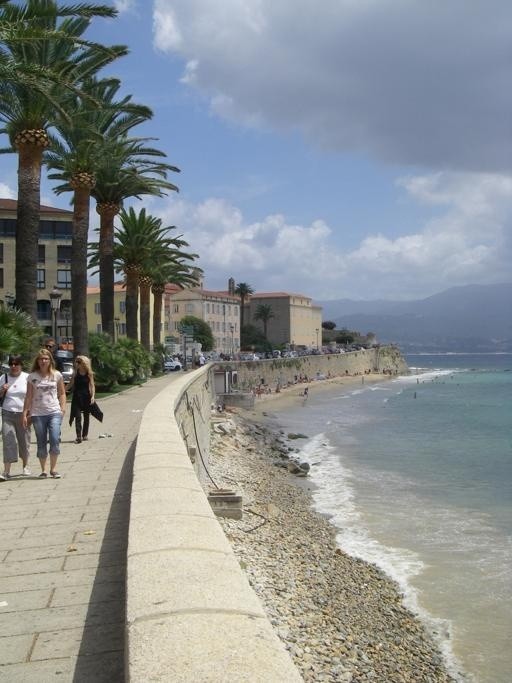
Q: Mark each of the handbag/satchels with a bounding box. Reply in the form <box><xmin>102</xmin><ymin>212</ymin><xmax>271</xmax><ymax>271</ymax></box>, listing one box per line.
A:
<box><xmin>0</xmin><ymin>372</ymin><xmax>7</xmax><ymax>406</ymax></box>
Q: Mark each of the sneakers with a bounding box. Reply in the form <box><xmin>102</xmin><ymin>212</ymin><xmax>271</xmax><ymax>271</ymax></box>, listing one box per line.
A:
<box><xmin>83</xmin><ymin>433</ymin><xmax>87</xmax><ymax>439</ymax></box>
<box><xmin>0</xmin><ymin>471</ymin><xmax>10</xmax><ymax>480</ymax></box>
<box><xmin>23</xmin><ymin>464</ymin><xmax>30</xmax><ymax>475</ymax></box>
<box><xmin>39</xmin><ymin>472</ymin><xmax>46</xmax><ymax>478</ymax></box>
<box><xmin>76</xmin><ymin>437</ymin><xmax>81</xmax><ymax>443</ymax></box>
<box><xmin>49</xmin><ymin>471</ymin><xmax>60</xmax><ymax>477</ymax></box>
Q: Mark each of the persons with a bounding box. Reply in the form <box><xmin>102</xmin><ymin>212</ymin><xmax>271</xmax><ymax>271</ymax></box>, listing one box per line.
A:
<box><xmin>44</xmin><ymin>337</ymin><xmax>64</xmax><ymax>443</ymax></box>
<box><xmin>63</xmin><ymin>354</ymin><xmax>96</xmax><ymax>443</ymax></box>
<box><xmin>303</xmin><ymin>387</ymin><xmax>308</xmax><ymax>400</ymax></box>
<box><xmin>198</xmin><ymin>353</ymin><xmax>205</xmax><ymax>366</ymax></box>
<box><xmin>216</xmin><ymin>401</ymin><xmax>222</xmax><ymax>413</ymax></box>
<box><xmin>0</xmin><ymin>351</ymin><xmax>31</xmax><ymax>481</ymax></box>
<box><xmin>19</xmin><ymin>348</ymin><xmax>66</xmax><ymax>480</ymax></box>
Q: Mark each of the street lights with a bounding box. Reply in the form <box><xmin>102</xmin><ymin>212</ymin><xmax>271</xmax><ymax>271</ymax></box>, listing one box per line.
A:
<box><xmin>230</xmin><ymin>325</ymin><xmax>235</xmax><ymax>354</ymax></box>
<box><xmin>316</xmin><ymin>327</ymin><xmax>320</xmax><ymax>353</ymax></box>
<box><xmin>114</xmin><ymin>317</ymin><xmax>121</xmax><ymax>339</ymax></box>
<box><xmin>49</xmin><ymin>285</ymin><xmax>62</xmax><ymax>370</ymax></box>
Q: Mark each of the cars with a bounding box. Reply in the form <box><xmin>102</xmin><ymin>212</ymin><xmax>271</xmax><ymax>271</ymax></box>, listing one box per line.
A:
<box><xmin>186</xmin><ymin>343</ymin><xmax>360</xmax><ymax>363</ymax></box>
<box><xmin>57</xmin><ymin>361</ymin><xmax>74</xmax><ymax>384</ymax></box>
<box><xmin>163</xmin><ymin>355</ymin><xmax>182</xmax><ymax>370</ymax></box>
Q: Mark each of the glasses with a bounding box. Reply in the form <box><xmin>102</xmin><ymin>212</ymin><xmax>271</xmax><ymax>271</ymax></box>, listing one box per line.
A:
<box><xmin>45</xmin><ymin>344</ymin><xmax>53</xmax><ymax>348</ymax></box>
<box><xmin>10</xmin><ymin>362</ymin><xmax>19</xmax><ymax>365</ymax></box>
<box><xmin>76</xmin><ymin>360</ymin><xmax>81</xmax><ymax>364</ymax></box>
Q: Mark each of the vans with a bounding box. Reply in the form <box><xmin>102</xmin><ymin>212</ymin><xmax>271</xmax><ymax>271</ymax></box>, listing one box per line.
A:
<box><xmin>54</xmin><ymin>350</ymin><xmax>74</xmax><ymax>367</ymax></box>
